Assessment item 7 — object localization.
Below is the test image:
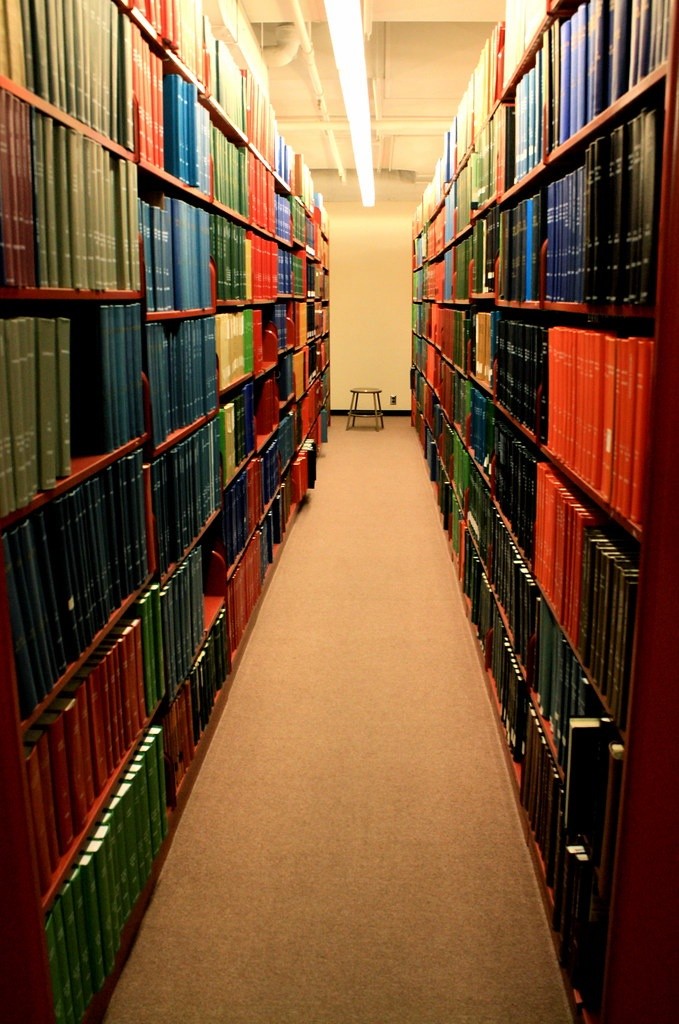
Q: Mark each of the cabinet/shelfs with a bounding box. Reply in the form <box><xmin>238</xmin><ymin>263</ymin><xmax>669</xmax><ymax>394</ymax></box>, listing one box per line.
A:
<box><xmin>0</xmin><ymin>0</ymin><xmax>331</xmax><ymax>1024</ymax></box>
<box><xmin>411</xmin><ymin>0</ymin><xmax>678</xmax><ymax>1024</ymax></box>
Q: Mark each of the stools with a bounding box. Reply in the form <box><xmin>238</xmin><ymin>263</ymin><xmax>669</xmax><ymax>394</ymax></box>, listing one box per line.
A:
<box><xmin>345</xmin><ymin>387</ymin><xmax>385</xmax><ymax>432</ymax></box>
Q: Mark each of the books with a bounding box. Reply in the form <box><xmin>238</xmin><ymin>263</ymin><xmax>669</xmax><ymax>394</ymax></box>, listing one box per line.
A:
<box><xmin>0</xmin><ymin>3</ymin><xmax>331</xmax><ymax>1024</ymax></box>
<box><xmin>411</xmin><ymin>1</ymin><xmax>679</xmax><ymax>1023</ymax></box>
